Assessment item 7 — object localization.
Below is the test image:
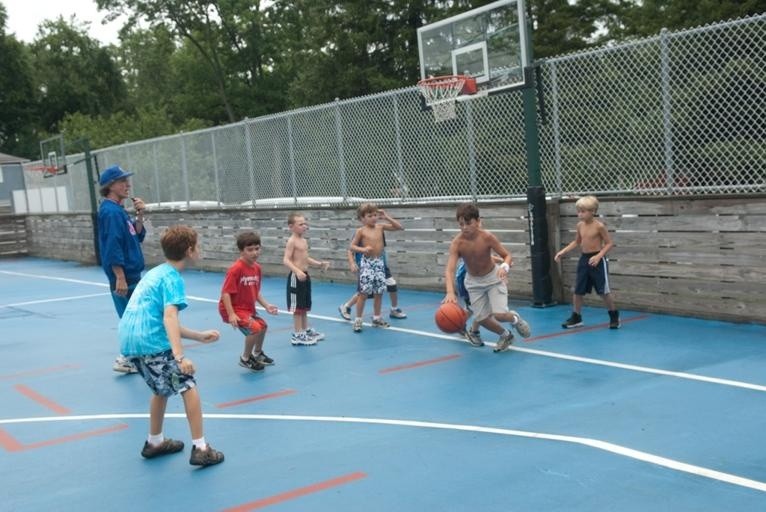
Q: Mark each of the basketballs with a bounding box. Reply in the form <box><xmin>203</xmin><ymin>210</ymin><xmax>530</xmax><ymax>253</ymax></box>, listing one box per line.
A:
<box><xmin>435</xmin><ymin>301</ymin><xmax>466</xmax><ymax>332</ymax></box>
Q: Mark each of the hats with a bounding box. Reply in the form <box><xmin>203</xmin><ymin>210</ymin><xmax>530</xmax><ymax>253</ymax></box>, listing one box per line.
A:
<box><xmin>100</xmin><ymin>166</ymin><xmax>134</xmax><ymax>186</ymax></box>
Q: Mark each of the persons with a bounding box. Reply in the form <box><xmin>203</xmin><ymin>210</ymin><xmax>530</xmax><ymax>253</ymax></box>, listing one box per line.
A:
<box><xmin>281</xmin><ymin>213</ymin><xmax>331</xmax><ymax>345</ymax></box>
<box><xmin>440</xmin><ymin>203</ymin><xmax>532</xmax><ymax>352</ymax></box>
<box><xmin>337</xmin><ymin>230</ymin><xmax>407</xmax><ymax>320</ymax></box>
<box><xmin>553</xmin><ymin>195</ymin><xmax>622</xmax><ymax>330</ymax></box>
<box><xmin>455</xmin><ymin>255</ymin><xmax>513</xmax><ymax>347</ymax></box>
<box><xmin>94</xmin><ymin>163</ymin><xmax>148</xmax><ymax>377</ymax></box>
<box><xmin>350</xmin><ymin>201</ymin><xmax>403</xmax><ymax>332</ymax></box>
<box><xmin>116</xmin><ymin>224</ymin><xmax>226</xmax><ymax>468</ymax></box>
<box><xmin>216</xmin><ymin>231</ymin><xmax>279</xmax><ymax>372</ymax></box>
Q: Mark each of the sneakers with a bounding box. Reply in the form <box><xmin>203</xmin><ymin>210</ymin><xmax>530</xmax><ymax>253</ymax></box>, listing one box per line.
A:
<box><xmin>371</xmin><ymin>318</ymin><xmax>390</xmax><ymax>328</ymax></box>
<box><xmin>253</xmin><ymin>351</ymin><xmax>274</xmax><ymax>365</ymax></box>
<box><xmin>353</xmin><ymin>319</ymin><xmax>362</xmax><ymax>331</ymax></box>
<box><xmin>190</xmin><ymin>443</ymin><xmax>224</xmax><ymax>464</ymax></box>
<box><xmin>113</xmin><ymin>357</ymin><xmax>139</xmax><ymax>372</ymax></box>
<box><xmin>493</xmin><ymin>330</ymin><xmax>515</xmax><ymax>352</ymax></box>
<box><xmin>464</xmin><ymin>328</ymin><xmax>484</xmax><ymax>347</ymax></box>
<box><xmin>510</xmin><ymin>311</ymin><xmax>531</xmax><ymax>339</ymax></box>
<box><xmin>238</xmin><ymin>354</ymin><xmax>264</xmax><ymax>370</ymax></box>
<box><xmin>337</xmin><ymin>304</ymin><xmax>351</xmax><ymax>320</ymax></box>
<box><xmin>459</xmin><ymin>324</ymin><xmax>468</xmax><ymax>338</ymax></box>
<box><xmin>389</xmin><ymin>308</ymin><xmax>407</xmax><ymax>319</ymax></box>
<box><xmin>304</xmin><ymin>328</ymin><xmax>325</xmax><ymax>340</ymax></box>
<box><xmin>608</xmin><ymin>310</ymin><xmax>620</xmax><ymax>329</ymax></box>
<box><xmin>561</xmin><ymin>312</ymin><xmax>584</xmax><ymax>329</ymax></box>
<box><xmin>141</xmin><ymin>438</ymin><xmax>185</xmax><ymax>458</ymax></box>
<box><xmin>291</xmin><ymin>332</ymin><xmax>317</xmax><ymax>345</ymax></box>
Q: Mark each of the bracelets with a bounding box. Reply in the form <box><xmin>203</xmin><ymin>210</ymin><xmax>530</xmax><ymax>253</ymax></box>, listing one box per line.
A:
<box><xmin>500</xmin><ymin>263</ymin><xmax>511</xmax><ymax>273</ymax></box>
